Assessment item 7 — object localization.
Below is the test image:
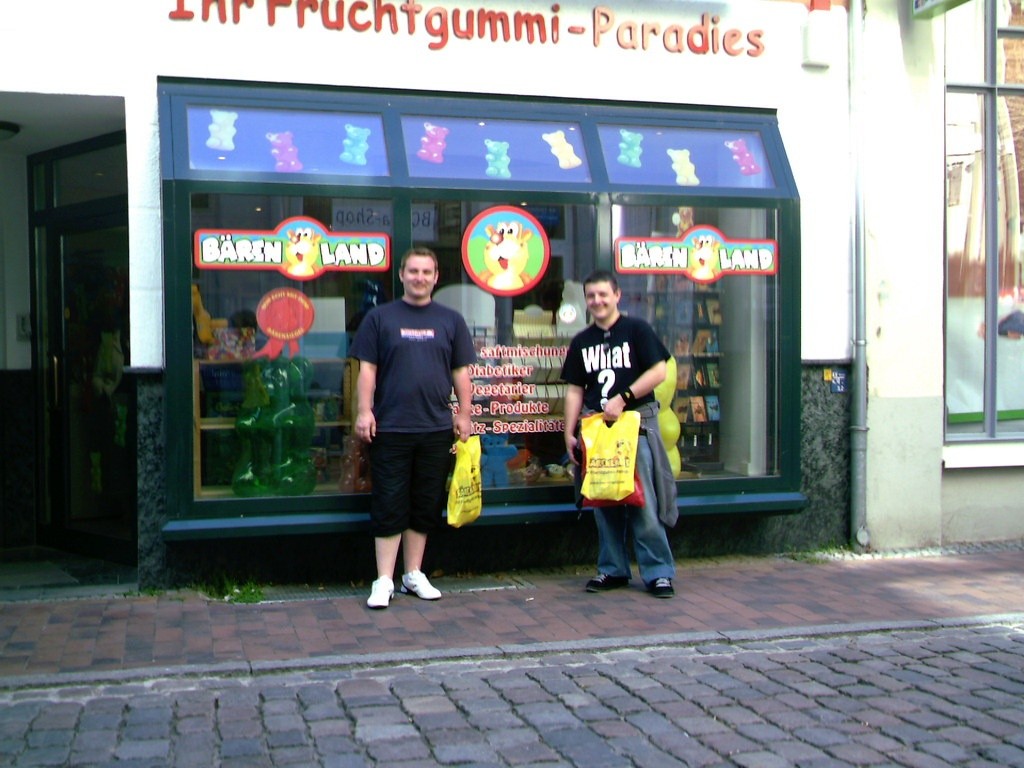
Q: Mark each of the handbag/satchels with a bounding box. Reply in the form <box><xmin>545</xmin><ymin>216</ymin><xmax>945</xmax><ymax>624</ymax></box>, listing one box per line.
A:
<box><xmin>579</xmin><ymin>413</ymin><xmax>645</xmax><ymax>508</ymax></box>
<box><xmin>581</xmin><ymin>411</ymin><xmax>641</xmax><ymax>501</ymax></box>
<box><xmin>446</xmin><ymin>434</ymin><xmax>483</xmax><ymax>528</ymax></box>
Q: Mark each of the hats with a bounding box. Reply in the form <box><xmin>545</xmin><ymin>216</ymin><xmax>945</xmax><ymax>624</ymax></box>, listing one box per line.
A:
<box><xmin>228</xmin><ymin>310</ymin><xmax>257</xmax><ymax>328</ymax></box>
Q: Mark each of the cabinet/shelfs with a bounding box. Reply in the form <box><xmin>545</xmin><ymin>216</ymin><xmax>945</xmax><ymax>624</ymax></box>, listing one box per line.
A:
<box><xmin>193</xmin><ymin>356</ymin><xmax>356</xmax><ymax>495</ymax></box>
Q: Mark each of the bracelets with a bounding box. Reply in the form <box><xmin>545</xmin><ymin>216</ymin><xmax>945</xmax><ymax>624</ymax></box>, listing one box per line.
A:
<box><xmin>619</xmin><ymin>387</ymin><xmax>636</xmax><ymax>405</ymax></box>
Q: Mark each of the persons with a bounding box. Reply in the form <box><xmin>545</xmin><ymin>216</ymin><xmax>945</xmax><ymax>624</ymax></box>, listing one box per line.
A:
<box><xmin>556</xmin><ymin>269</ymin><xmax>675</xmax><ymax>600</ymax></box>
<box><xmin>348</xmin><ymin>247</ymin><xmax>478</xmax><ymax>607</ymax></box>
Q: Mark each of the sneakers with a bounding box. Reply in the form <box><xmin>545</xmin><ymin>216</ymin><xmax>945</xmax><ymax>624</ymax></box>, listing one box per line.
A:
<box><xmin>367</xmin><ymin>576</ymin><xmax>395</xmax><ymax>608</ymax></box>
<box><xmin>400</xmin><ymin>571</ymin><xmax>442</xmax><ymax>599</ymax></box>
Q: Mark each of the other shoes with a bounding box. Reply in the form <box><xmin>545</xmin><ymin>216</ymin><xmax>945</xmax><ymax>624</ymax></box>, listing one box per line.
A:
<box><xmin>648</xmin><ymin>577</ymin><xmax>674</xmax><ymax>598</ymax></box>
<box><xmin>585</xmin><ymin>573</ymin><xmax>629</xmax><ymax>592</ymax></box>
